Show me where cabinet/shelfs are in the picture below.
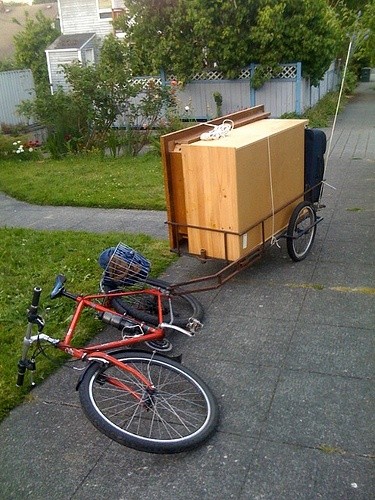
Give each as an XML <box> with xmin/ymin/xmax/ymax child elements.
<box><xmin>160</xmin><ymin>104</ymin><xmax>309</xmax><ymax>261</ymax></box>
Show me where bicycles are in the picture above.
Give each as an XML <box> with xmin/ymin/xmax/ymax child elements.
<box><xmin>16</xmin><ymin>241</ymin><xmax>223</xmax><ymax>457</ymax></box>
